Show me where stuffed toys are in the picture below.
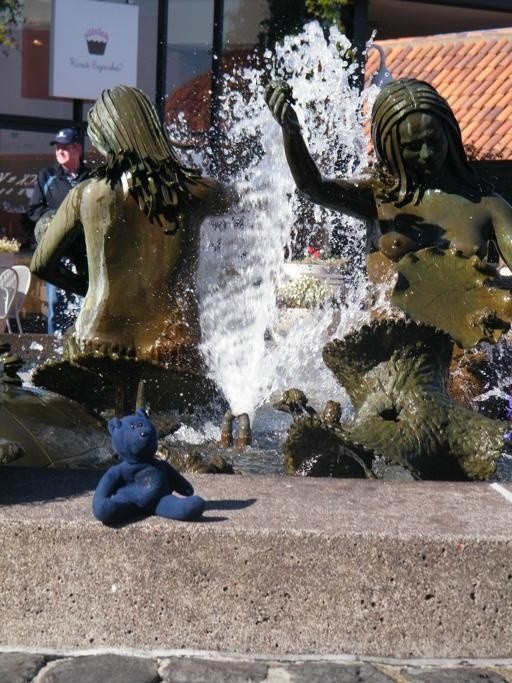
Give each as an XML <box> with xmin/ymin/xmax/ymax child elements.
<box><xmin>89</xmin><ymin>405</ymin><xmax>206</xmax><ymax>527</ymax></box>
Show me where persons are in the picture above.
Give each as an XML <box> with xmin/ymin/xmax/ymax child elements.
<box><xmin>24</xmin><ymin>126</ymin><xmax>100</xmax><ymax>335</ymax></box>
<box><xmin>28</xmin><ymin>82</ymin><xmax>235</xmax><ymax>373</ymax></box>
<box><xmin>261</xmin><ymin>66</ymin><xmax>511</xmax><ymax>320</ymax></box>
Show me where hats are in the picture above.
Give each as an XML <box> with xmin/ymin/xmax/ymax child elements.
<box><xmin>50</xmin><ymin>128</ymin><xmax>80</xmax><ymax>146</ymax></box>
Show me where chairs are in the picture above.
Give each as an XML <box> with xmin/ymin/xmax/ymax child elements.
<box><xmin>0</xmin><ymin>264</ymin><xmax>32</xmax><ymax>335</ymax></box>
